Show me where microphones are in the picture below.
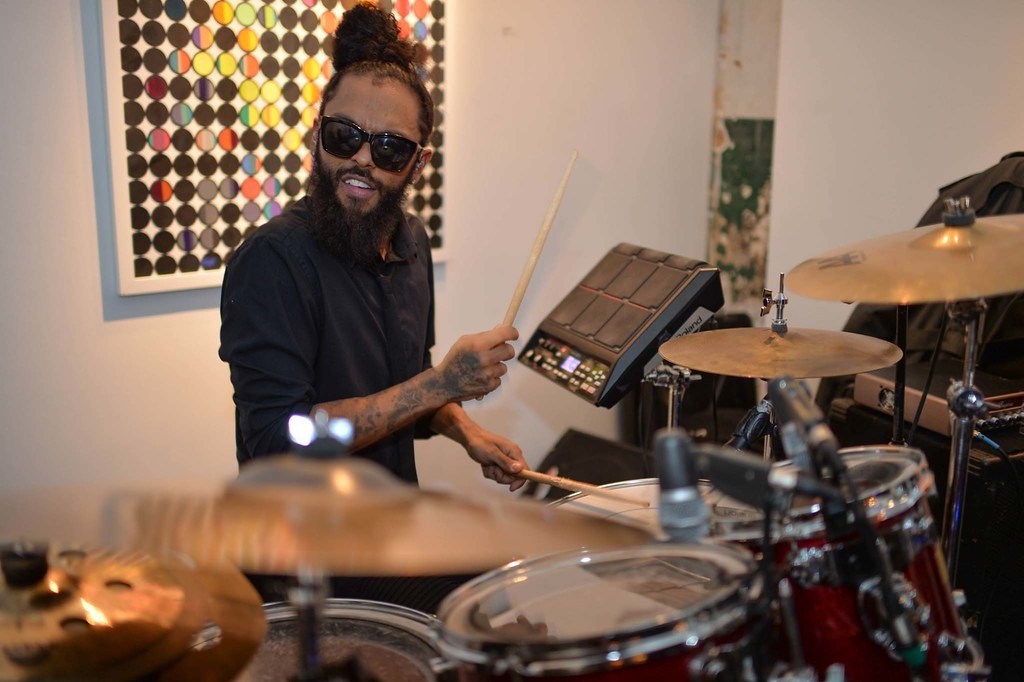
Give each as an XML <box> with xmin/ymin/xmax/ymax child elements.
<box><xmin>656</xmin><ymin>430</ymin><xmax>711</xmax><ymax>538</ymax></box>
<box><xmin>770</xmin><ymin>374</ymin><xmax>843</xmax><ymax>470</ymax></box>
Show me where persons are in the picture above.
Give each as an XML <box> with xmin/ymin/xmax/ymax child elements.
<box><xmin>217</xmin><ymin>0</ymin><xmax>533</xmax><ymax>499</ymax></box>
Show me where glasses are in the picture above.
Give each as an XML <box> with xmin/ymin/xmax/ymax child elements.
<box><xmin>320</xmin><ymin>116</ymin><xmax>420</xmax><ymax>173</ymax></box>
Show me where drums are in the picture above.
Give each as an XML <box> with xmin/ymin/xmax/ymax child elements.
<box><xmin>232</xmin><ymin>445</ymin><xmax>992</xmax><ymax>681</ymax></box>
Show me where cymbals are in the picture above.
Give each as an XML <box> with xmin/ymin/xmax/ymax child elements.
<box><xmin>0</xmin><ymin>440</ymin><xmax>653</xmax><ymax>576</ymax></box>
<box><xmin>658</xmin><ymin>327</ymin><xmax>902</xmax><ymax>377</ymax></box>
<box><xmin>785</xmin><ymin>213</ymin><xmax>1024</xmax><ymax>304</ymax></box>
<box><xmin>2</xmin><ymin>544</ymin><xmax>269</xmax><ymax>682</ymax></box>
